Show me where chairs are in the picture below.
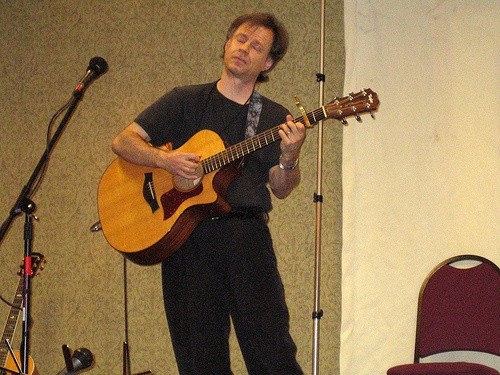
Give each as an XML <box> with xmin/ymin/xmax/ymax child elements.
<box><xmin>385</xmin><ymin>254</ymin><xmax>500</xmax><ymax>375</ymax></box>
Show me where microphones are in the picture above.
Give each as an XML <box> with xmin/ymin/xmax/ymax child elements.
<box><xmin>57</xmin><ymin>347</ymin><xmax>93</xmax><ymax>375</ymax></box>
<box><xmin>71</xmin><ymin>56</ymin><xmax>108</xmax><ymax>95</ymax></box>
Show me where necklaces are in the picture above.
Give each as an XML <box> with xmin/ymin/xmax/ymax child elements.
<box><xmin>192</xmin><ymin>75</ymin><xmax>255</xmax><ymax>135</ymax></box>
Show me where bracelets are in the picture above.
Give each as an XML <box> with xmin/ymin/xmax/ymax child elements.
<box><xmin>280</xmin><ymin>158</ymin><xmax>299</xmax><ymax>170</ymax></box>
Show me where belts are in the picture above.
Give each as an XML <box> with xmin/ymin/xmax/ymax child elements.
<box><xmin>233</xmin><ymin>210</ymin><xmax>260</xmax><ymax>219</ymax></box>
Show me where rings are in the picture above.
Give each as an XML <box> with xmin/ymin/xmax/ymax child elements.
<box><xmin>286</xmin><ymin>131</ymin><xmax>291</xmax><ymax>135</ymax></box>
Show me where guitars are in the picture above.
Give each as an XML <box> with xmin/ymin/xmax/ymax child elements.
<box><xmin>97</xmin><ymin>87</ymin><xmax>382</xmax><ymax>266</ymax></box>
<box><xmin>0</xmin><ymin>252</ymin><xmax>47</xmax><ymax>375</ymax></box>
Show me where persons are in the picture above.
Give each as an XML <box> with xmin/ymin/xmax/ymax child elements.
<box><xmin>111</xmin><ymin>13</ymin><xmax>304</xmax><ymax>375</ymax></box>
